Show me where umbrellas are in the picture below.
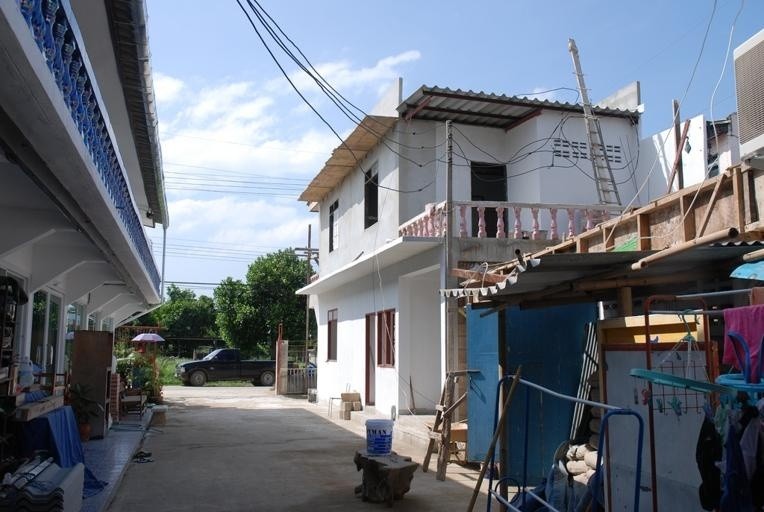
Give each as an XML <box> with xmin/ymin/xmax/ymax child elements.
<box><xmin>130</xmin><ymin>333</ymin><xmax>165</xmax><ymax>343</ymax></box>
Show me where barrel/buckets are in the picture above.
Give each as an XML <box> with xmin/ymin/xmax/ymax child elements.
<box><xmin>366</xmin><ymin>419</ymin><xmax>394</xmax><ymax>457</ymax></box>
<box><xmin>20</xmin><ymin>356</ymin><xmax>34</xmax><ymax>388</ymax></box>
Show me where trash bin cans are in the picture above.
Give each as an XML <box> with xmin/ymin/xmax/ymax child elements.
<box><xmin>290</xmin><ymin>364</ymin><xmax>301</xmax><ymax>395</ymax></box>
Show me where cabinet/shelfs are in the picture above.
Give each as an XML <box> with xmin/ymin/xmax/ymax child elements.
<box><xmin>69</xmin><ymin>329</ymin><xmax>115</xmax><ymax>441</ymax></box>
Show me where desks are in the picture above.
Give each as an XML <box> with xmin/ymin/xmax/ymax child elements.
<box><xmin>121</xmin><ymin>394</ymin><xmax>149</xmax><ymax>421</ymax></box>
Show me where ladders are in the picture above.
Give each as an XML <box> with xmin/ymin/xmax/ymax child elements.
<box><xmin>422</xmin><ymin>370</ymin><xmax>469</xmax><ymax>479</ymax></box>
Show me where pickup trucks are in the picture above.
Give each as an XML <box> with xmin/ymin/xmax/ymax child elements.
<box><xmin>174</xmin><ymin>348</ymin><xmax>300</xmax><ymax>387</ymax></box>
